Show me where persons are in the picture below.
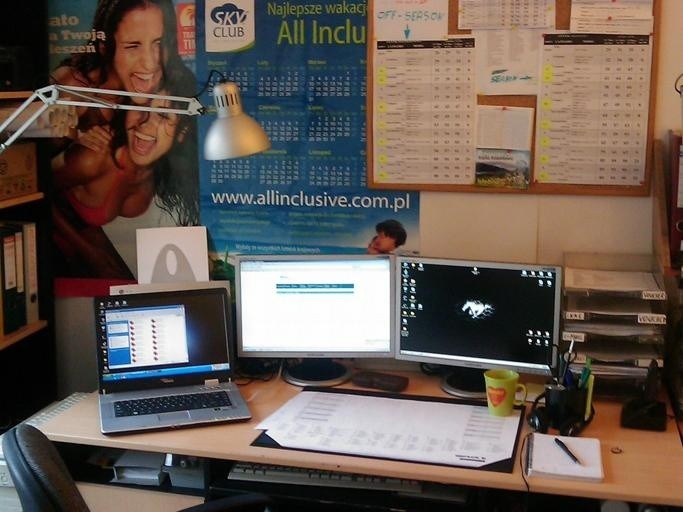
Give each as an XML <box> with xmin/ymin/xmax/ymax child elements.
<box><xmin>366</xmin><ymin>236</ymin><xmax>383</xmax><ymax>255</ymax></box>
<box><xmin>374</xmin><ymin>218</ymin><xmax>415</xmax><ymax>257</ymax></box>
<box><xmin>46</xmin><ymin>0</ymin><xmax>205</xmax><ymax>165</ymax></box>
<box><xmin>45</xmin><ymin>70</ymin><xmax>204</xmax><ymax>279</ymax></box>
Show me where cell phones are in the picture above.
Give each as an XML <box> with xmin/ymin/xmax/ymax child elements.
<box><xmin>351</xmin><ymin>370</ymin><xmax>409</xmax><ymax>392</ymax></box>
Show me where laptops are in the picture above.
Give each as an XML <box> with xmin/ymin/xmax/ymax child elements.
<box><xmin>94</xmin><ymin>286</ymin><xmax>253</xmax><ymax>436</ymax></box>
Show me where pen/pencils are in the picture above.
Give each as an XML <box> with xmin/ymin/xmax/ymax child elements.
<box><xmin>555</xmin><ymin>438</ymin><xmax>582</xmax><ymax>466</ymax></box>
<box><xmin>561</xmin><ymin>339</ymin><xmax>595</xmax><ymax>421</ymax></box>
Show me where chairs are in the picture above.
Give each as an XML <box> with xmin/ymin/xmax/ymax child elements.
<box><xmin>0</xmin><ymin>422</ymin><xmax>279</xmax><ymax>512</ymax></box>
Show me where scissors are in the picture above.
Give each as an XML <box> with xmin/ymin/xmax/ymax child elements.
<box><xmin>546</xmin><ymin>344</ymin><xmax>577</xmax><ymax>385</ymax></box>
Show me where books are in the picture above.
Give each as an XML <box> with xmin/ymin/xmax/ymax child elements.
<box><xmin>0</xmin><ymin>223</ymin><xmax>26</xmax><ymax>327</ymax></box>
<box><xmin>524</xmin><ymin>431</ymin><xmax>604</xmax><ymax>482</ymax></box>
<box><xmin>0</xmin><ymin>233</ymin><xmax>15</xmax><ymax>336</ymax></box>
<box><xmin>2</xmin><ymin>218</ymin><xmax>41</xmax><ymax>325</ymax></box>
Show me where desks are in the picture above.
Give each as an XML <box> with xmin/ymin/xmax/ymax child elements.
<box><xmin>34</xmin><ymin>367</ymin><xmax>683</xmax><ymax>512</ymax></box>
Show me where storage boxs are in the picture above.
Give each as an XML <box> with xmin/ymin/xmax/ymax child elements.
<box><xmin>0</xmin><ymin>141</ymin><xmax>40</xmax><ymax>202</ymax></box>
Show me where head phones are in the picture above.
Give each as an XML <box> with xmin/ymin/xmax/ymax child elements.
<box><xmin>527</xmin><ymin>390</ymin><xmax>596</xmax><ymax>437</ymax></box>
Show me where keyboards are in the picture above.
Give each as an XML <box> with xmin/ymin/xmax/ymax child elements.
<box><xmin>227</xmin><ymin>462</ymin><xmax>423</xmax><ymax>494</ymax></box>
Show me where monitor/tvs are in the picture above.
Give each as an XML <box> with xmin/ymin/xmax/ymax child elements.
<box><xmin>397</xmin><ymin>255</ymin><xmax>564</xmax><ymax>401</ymax></box>
<box><xmin>233</xmin><ymin>254</ymin><xmax>396</xmax><ymax>388</ymax></box>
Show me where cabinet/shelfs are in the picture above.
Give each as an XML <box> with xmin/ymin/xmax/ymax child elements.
<box><xmin>562</xmin><ymin>250</ymin><xmax>667</xmax><ymax>399</ymax></box>
<box><xmin>0</xmin><ymin>91</ymin><xmax>50</xmax><ymax>355</ymax></box>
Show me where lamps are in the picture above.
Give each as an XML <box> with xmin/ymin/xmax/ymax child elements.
<box><xmin>1</xmin><ymin>70</ymin><xmax>270</xmax><ymax>161</ymax></box>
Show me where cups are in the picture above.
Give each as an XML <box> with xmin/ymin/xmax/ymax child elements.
<box><xmin>481</xmin><ymin>368</ymin><xmax>526</xmax><ymax>416</ymax></box>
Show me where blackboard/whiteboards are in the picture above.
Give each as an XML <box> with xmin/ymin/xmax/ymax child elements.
<box><xmin>367</xmin><ymin>0</ymin><xmax>662</xmax><ymax>198</ymax></box>
